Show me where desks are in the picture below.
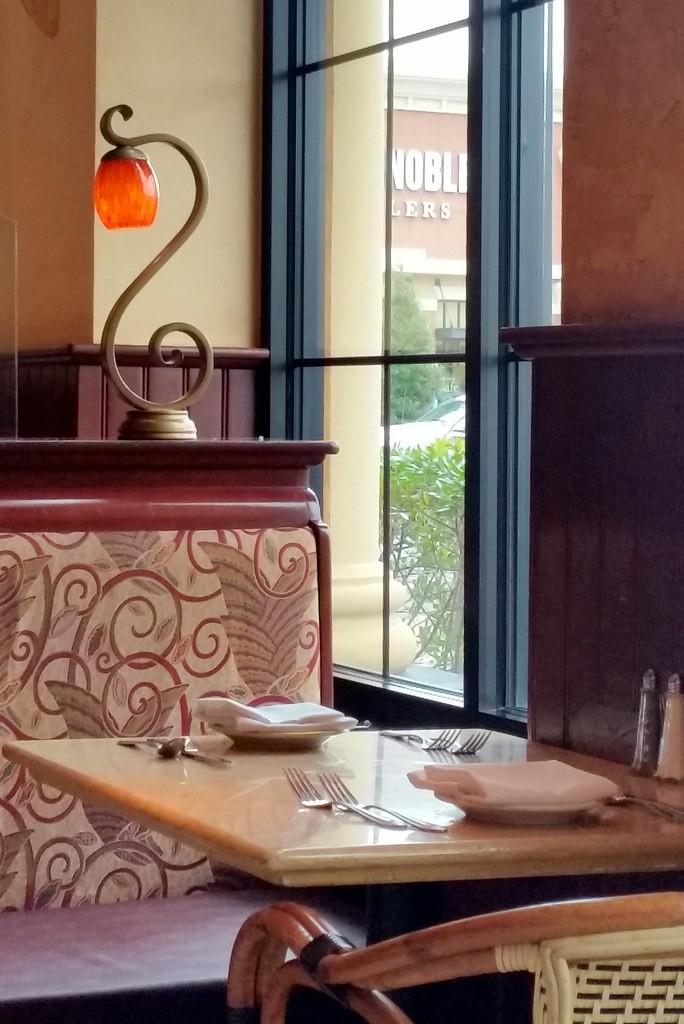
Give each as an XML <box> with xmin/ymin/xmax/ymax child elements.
<box><xmin>2</xmin><ymin>729</ymin><xmax>684</xmax><ymax>1024</ymax></box>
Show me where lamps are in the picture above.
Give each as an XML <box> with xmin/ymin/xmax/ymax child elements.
<box><xmin>92</xmin><ymin>105</ymin><xmax>213</xmax><ymax>439</ymax></box>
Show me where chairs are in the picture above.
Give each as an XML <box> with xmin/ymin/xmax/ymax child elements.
<box><xmin>226</xmin><ymin>890</ymin><xmax>684</xmax><ymax>1024</ymax></box>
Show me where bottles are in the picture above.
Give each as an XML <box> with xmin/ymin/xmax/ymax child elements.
<box><xmin>629</xmin><ymin>669</ymin><xmax>659</xmax><ymax>775</ymax></box>
<box><xmin>653</xmin><ymin>673</ymin><xmax>684</xmax><ymax>782</ymax></box>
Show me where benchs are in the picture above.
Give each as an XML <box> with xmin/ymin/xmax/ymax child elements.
<box><xmin>0</xmin><ymin>486</ymin><xmax>381</xmax><ymax>1024</ymax></box>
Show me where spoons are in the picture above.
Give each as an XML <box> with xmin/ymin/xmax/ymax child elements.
<box><xmin>117</xmin><ymin>737</ymin><xmax>191</xmax><ymax>757</ymax></box>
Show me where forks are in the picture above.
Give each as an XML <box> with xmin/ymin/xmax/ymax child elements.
<box><xmin>284</xmin><ymin>767</ymin><xmax>407</xmax><ymax>830</ymax></box>
<box><xmin>379</xmin><ymin>730</ymin><xmax>461</xmax><ymax>750</ymax></box>
<box><xmin>406</xmin><ymin>733</ymin><xmax>491</xmax><ymax>754</ymax></box>
<box><xmin>318</xmin><ymin>772</ymin><xmax>446</xmax><ymax>833</ymax></box>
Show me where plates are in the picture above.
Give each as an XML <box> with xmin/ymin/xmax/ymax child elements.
<box><xmin>207</xmin><ymin>722</ymin><xmax>350</xmax><ymax>748</ymax></box>
<box><xmin>433</xmin><ymin>790</ymin><xmax>602</xmax><ymax>825</ymax></box>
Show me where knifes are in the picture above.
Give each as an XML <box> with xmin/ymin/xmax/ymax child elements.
<box><xmin>148</xmin><ymin>738</ymin><xmax>231</xmax><ymax>764</ymax></box>
